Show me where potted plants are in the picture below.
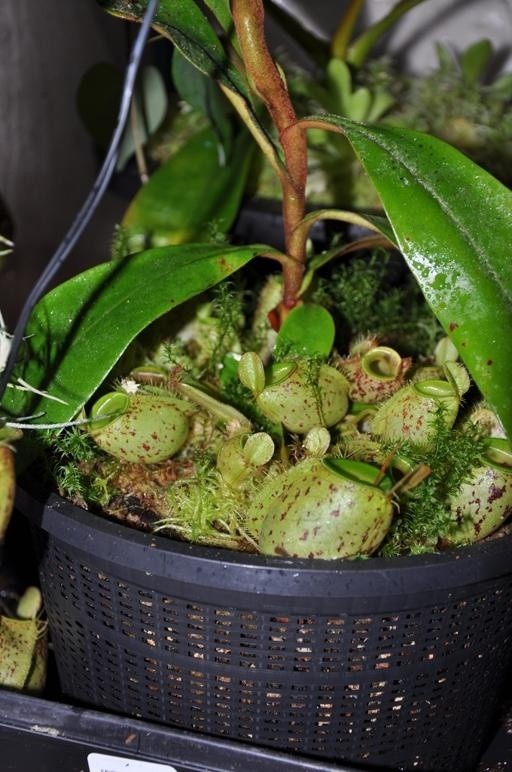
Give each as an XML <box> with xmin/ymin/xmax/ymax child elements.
<box><xmin>1</xmin><ymin>1</ymin><xmax>511</xmax><ymax>771</ymax></box>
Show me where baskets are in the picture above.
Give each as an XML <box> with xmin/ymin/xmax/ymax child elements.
<box><xmin>11</xmin><ymin>449</ymin><xmax>512</xmax><ymax>772</ymax></box>
